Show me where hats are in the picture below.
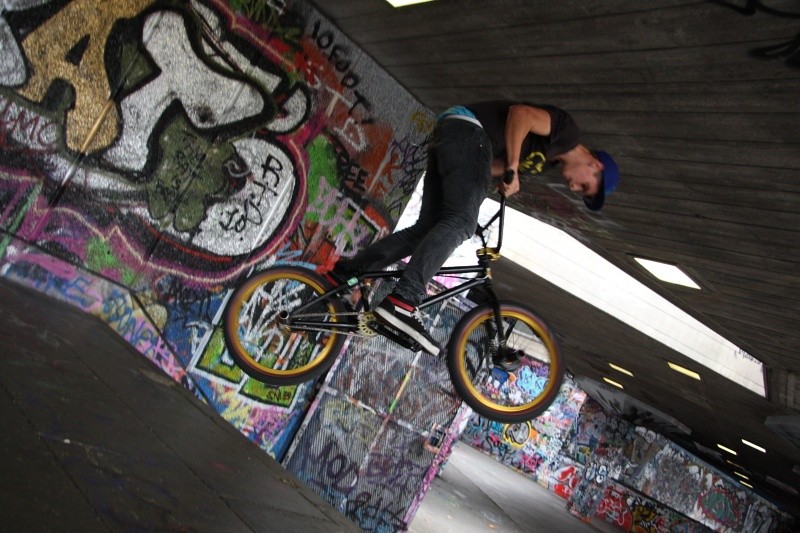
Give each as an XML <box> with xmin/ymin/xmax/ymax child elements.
<box><xmin>583</xmin><ymin>151</ymin><xmax>618</xmax><ymax>212</ymax></box>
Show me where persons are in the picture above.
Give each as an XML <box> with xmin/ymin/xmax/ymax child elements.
<box><xmin>325</xmin><ymin>99</ymin><xmax>619</xmax><ymax>355</ymax></box>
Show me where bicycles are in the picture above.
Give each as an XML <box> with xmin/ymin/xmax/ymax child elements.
<box><xmin>221</xmin><ymin>169</ymin><xmax>566</xmax><ymax>424</ymax></box>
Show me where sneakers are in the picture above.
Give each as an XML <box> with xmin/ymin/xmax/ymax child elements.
<box><xmin>314</xmin><ymin>272</ymin><xmax>354</xmax><ymax>306</ymax></box>
<box><xmin>373</xmin><ymin>293</ymin><xmax>441</xmax><ymax>357</ymax></box>
